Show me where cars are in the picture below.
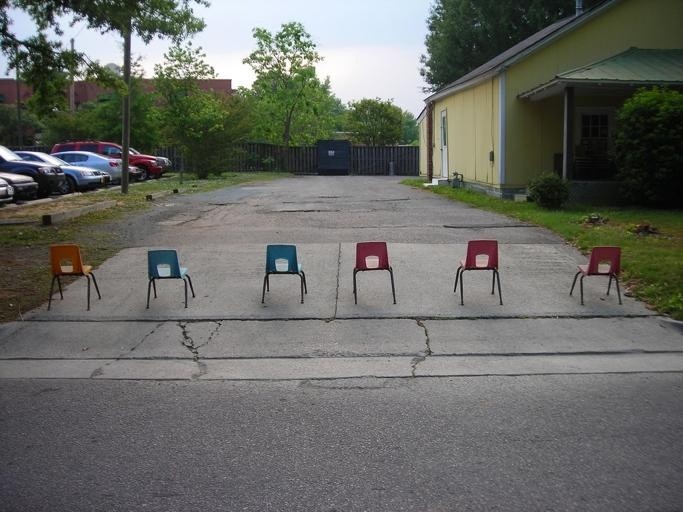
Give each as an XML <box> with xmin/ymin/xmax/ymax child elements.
<box><xmin>0</xmin><ymin>144</ymin><xmax>141</xmax><ymax>203</ymax></box>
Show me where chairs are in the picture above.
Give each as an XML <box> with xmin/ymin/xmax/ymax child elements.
<box><xmin>352</xmin><ymin>241</ymin><xmax>396</xmax><ymax>304</ymax></box>
<box><xmin>146</xmin><ymin>249</ymin><xmax>195</xmax><ymax>309</ymax></box>
<box><xmin>454</xmin><ymin>240</ymin><xmax>503</xmax><ymax>305</ymax></box>
<box><xmin>570</xmin><ymin>246</ymin><xmax>622</xmax><ymax>305</ymax></box>
<box><xmin>47</xmin><ymin>242</ymin><xmax>101</xmax><ymax>312</ymax></box>
<box><xmin>261</xmin><ymin>244</ymin><xmax>307</xmax><ymax>303</ymax></box>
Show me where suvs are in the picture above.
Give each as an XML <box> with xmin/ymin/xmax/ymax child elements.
<box><xmin>127</xmin><ymin>147</ymin><xmax>173</xmax><ymax>178</ymax></box>
<box><xmin>51</xmin><ymin>141</ymin><xmax>161</xmax><ymax>181</ymax></box>
